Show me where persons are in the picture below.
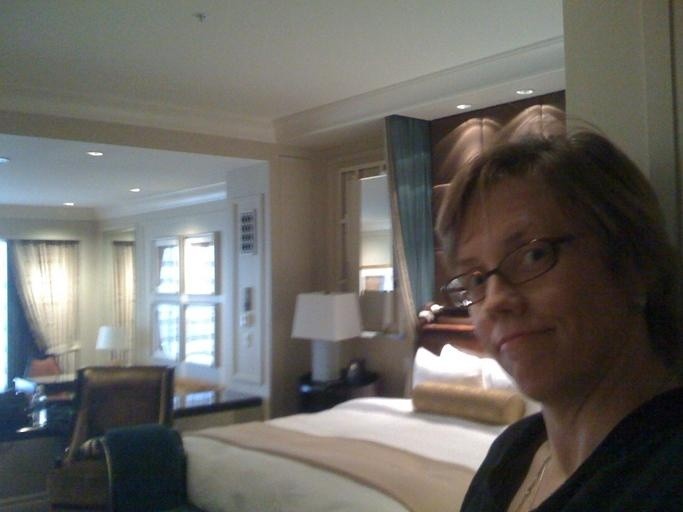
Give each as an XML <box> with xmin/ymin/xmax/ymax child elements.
<box><xmin>433</xmin><ymin>128</ymin><xmax>683</xmax><ymax>512</ymax></box>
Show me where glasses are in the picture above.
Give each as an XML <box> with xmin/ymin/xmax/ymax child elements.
<box><xmin>440</xmin><ymin>232</ymin><xmax>580</xmax><ymax>307</ymax></box>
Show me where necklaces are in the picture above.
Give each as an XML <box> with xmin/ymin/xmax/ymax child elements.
<box><xmin>515</xmin><ymin>456</ymin><xmax>554</xmax><ymax>512</ymax></box>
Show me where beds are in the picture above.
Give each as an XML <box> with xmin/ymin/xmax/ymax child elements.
<box><xmin>179</xmin><ymin>395</ymin><xmax>519</xmax><ymax>511</ymax></box>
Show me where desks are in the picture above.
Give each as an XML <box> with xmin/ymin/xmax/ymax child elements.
<box><xmin>10</xmin><ymin>374</ymin><xmax>76</xmax><ymax>427</ymax></box>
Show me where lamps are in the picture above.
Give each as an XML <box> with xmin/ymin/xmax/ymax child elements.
<box><xmin>94</xmin><ymin>323</ymin><xmax>131</xmax><ymax>362</ymax></box>
<box><xmin>289</xmin><ymin>291</ymin><xmax>363</xmax><ymax>384</ymax></box>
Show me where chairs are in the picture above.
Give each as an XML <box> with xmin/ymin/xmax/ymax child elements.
<box><xmin>99</xmin><ymin>421</ymin><xmax>186</xmax><ymax>512</ymax></box>
<box><xmin>43</xmin><ymin>363</ymin><xmax>187</xmax><ymax>512</ymax></box>
<box><xmin>21</xmin><ymin>354</ymin><xmax>76</xmax><ymax>422</ymax></box>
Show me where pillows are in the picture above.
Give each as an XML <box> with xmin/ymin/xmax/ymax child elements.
<box><xmin>412</xmin><ymin>342</ymin><xmax>541</xmax><ymax>419</ymax></box>
